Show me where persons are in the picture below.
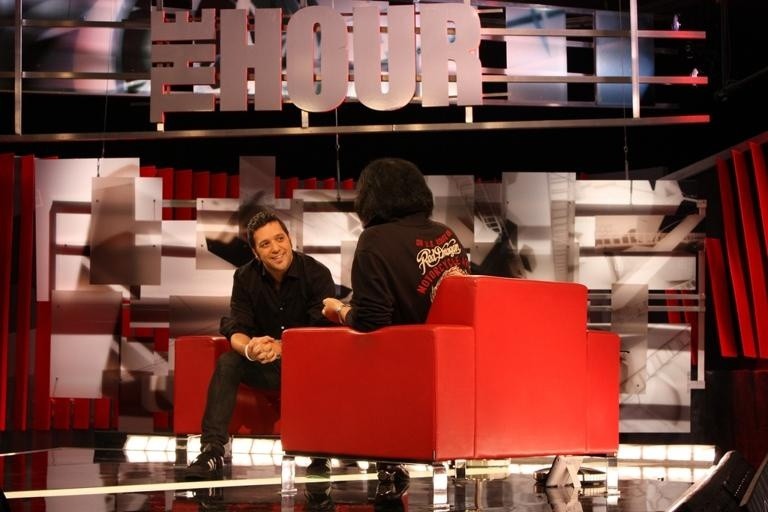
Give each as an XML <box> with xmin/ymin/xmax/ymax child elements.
<box><xmin>183</xmin><ymin>208</ymin><xmax>337</xmax><ymax>479</ymax></box>
<box><xmin>321</xmin><ymin>156</ymin><xmax>475</xmax><ymax>504</ymax></box>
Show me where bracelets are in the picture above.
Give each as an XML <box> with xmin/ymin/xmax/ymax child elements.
<box><xmin>337</xmin><ymin>304</ymin><xmax>352</xmax><ymax>328</ymax></box>
<box><xmin>244</xmin><ymin>344</ymin><xmax>255</xmax><ymax>362</ymax></box>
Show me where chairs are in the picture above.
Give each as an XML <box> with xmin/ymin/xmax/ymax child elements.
<box><xmin>174</xmin><ymin>334</ymin><xmax>280</xmax><ymax>467</ymax></box>
<box><xmin>279</xmin><ymin>274</ymin><xmax>620</xmax><ymax>512</ymax></box>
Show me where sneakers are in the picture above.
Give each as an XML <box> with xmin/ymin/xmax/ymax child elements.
<box><xmin>184</xmin><ymin>449</ymin><xmax>224</xmax><ymax>479</ymax></box>
<box><xmin>196</xmin><ymin>488</ymin><xmax>226</xmax><ymax>510</ymax></box>
<box><xmin>305</xmin><ymin>484</ymin><xmax>335</xmax><ymax>510</ymax></box>
<box><xmin>375</xmin><ymin>463</ymin><xmax>410</xmax><ymax>500</ymax></box>
<box><xmin>307</xmin><ymin>458</ymin><xmax>331</xmax><ymax>474</ymax></box>
